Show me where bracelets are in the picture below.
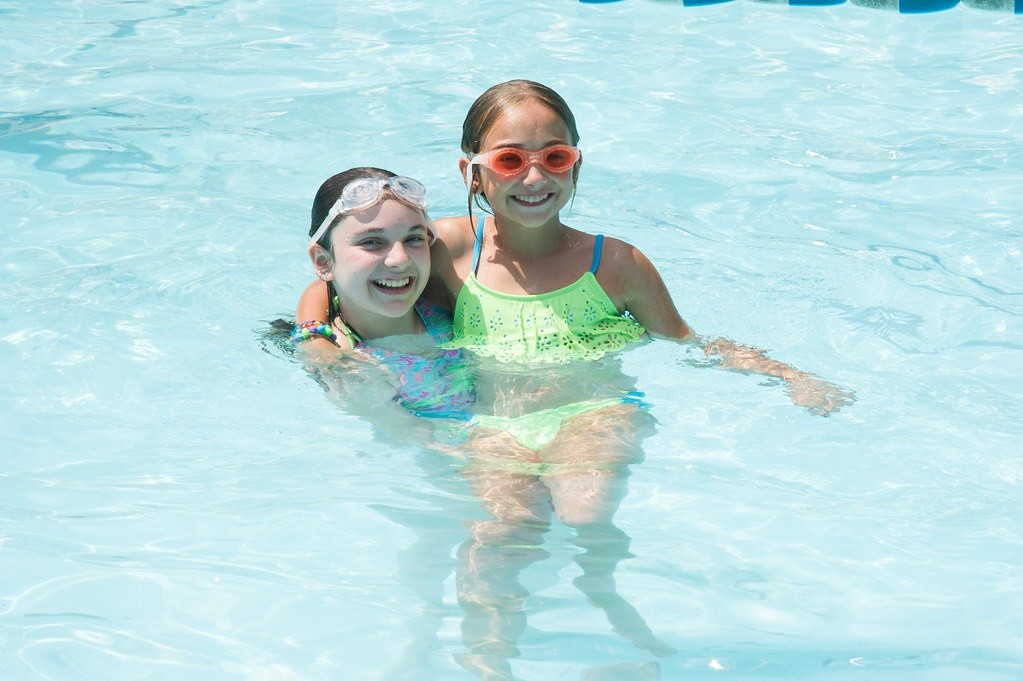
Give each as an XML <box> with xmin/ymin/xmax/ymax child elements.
<box><xmin>290</xmin><ymin>319</ymin><xmax>337</xmax><ymax>344</ymax></box>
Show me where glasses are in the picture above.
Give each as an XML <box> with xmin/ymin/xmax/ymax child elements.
<box><xmin>307</xmin><ymin>176</ymin><xmax>427</xmax><ymax>250</ymax></box>
<box><xmin>466</xmin><ymin>144</ymin><xmax>580</xmax><ymax>176</ymax></box>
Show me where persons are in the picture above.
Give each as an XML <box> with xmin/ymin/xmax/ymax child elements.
<box><xmin>296</xmin><ymin>78</ymin><xmax>687</xmax><ymax>357</ymax></box>
<box><xmin>307</xmin><ymin>169</ymin><xmax>454</xmax><ymax>349</ymax></box>
